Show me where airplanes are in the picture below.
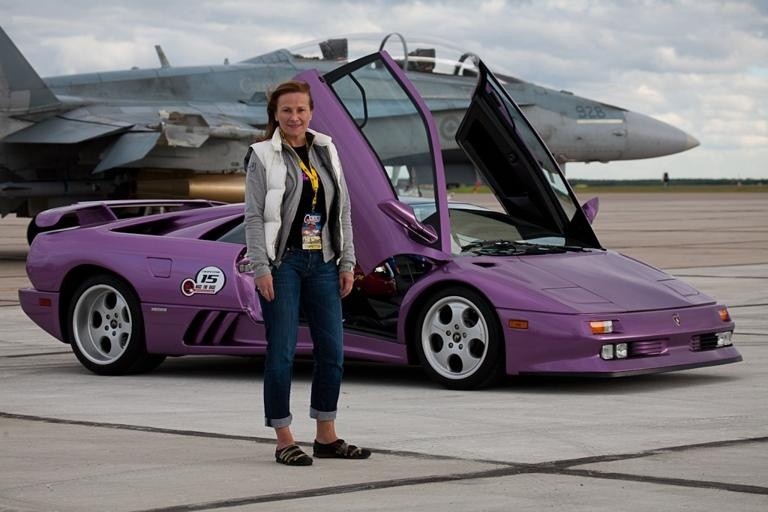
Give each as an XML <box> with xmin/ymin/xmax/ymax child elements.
<box><xmin>2</xmin><ymin>24</ymin><xmax>698</xmax><ymax>248</ymax></box>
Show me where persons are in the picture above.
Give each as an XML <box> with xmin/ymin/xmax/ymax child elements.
<box><xmin>663</xmin><ymin>172</ymin><xmax>669</xmax><ymax>188</ymax></box>
<box><xmin>242</xmin><ymin>81</ymin><xmax>371</xmax><ymax>466</ymax></box>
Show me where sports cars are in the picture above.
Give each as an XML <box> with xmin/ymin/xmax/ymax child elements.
<box><xmin>12</xmin><ymin>51</ymin><xmax>745</xmax><ymax>384</ymax></box>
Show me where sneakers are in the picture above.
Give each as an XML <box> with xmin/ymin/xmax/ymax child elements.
<box><xmin>276</xmin><ymin>445</ymin><xmax>312</xmax><ymax>466</ymax></box>
<box><xmin>314</xmin><ymin>438</ymin><xmax>370</xmax><ymax>460</ymax></box>
